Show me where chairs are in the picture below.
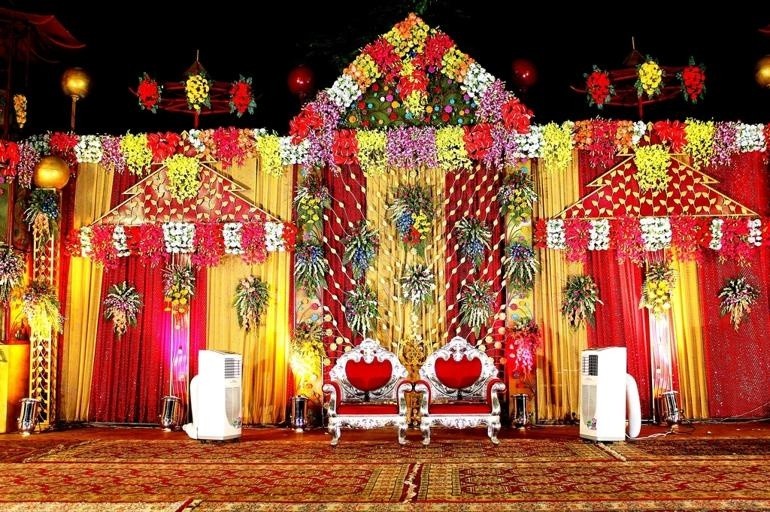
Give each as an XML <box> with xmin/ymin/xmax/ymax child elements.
<box><xmin>321</xmin><ymin>338</ymin><xmax>412</xmax><ymax>447</ymax></box>
<box><xmin>413</xmin><ymin>335</ymin><xmax>506</xmax><ymax>446</ymax></box>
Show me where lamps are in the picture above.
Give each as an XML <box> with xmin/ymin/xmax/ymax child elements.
<box><xmin>512</xmin><ymin>394</ymin><xmax>530</xmax><ymax>430</ymax></box>
<box><xmin>159</xmin><ymin>396</ymin><xmax>183</xmax><ymax>429</ymax></box>
<box><xmin>16</xmin><ymin>396</ymin><xmax>39</xmax><ymax>434</ymax></box>
<box><xmin>657</xmin><ymin>391</ymin><xmax>683</xmax><ymax>425</ymax></box>
<box><xmin>290</xmin><ymin>394</ymin><xmax>309</xmax><ymax>427</ymax></box>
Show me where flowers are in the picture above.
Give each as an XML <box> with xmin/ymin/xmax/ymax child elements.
<box><xmin>0</xmin><ymin>12</ymin><xmax>770</xmax><ymax>375</ymax></box>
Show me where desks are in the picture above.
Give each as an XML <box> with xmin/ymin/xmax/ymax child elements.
<box><xmin>1</xmin><ymin>343</ymin><xmax>30</xmax><ymax>434</ymax></box>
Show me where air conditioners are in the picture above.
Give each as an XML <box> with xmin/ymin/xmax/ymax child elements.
<box><xmin>579</xmin><ymin>344</ymin><xmax>643</xmax><ymax>442</ymax></box>
<box><xmin>188</xmin><ymin>349</ymin><xmax>244</xmax><ymax>440</ymax></box>
